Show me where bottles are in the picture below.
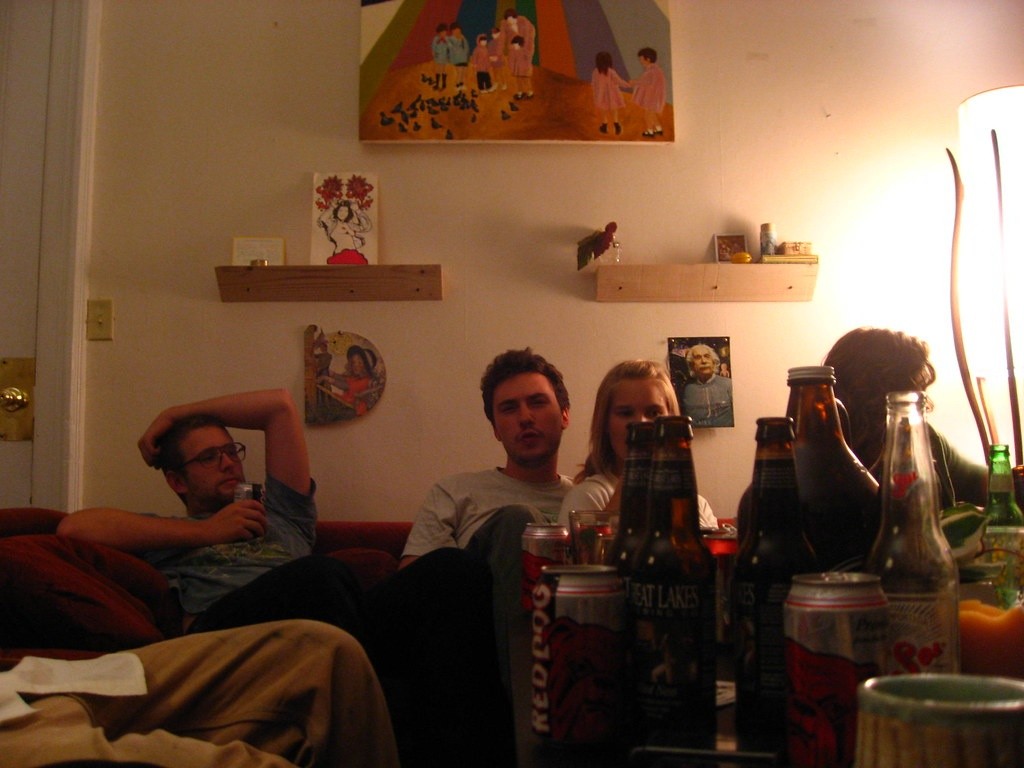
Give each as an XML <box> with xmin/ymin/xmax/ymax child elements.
<box><xmin>734</xmin><ymin>416</ymin><xmax>818</xmax><ymax>768</ymax></box>
<box><xmin>605</xmin><ymin>422</ymin><xmax>660</xmax><ymax>589</ymax></box>
<box><xmin>977</xmin><ymin>446</ymin><xmax>1024</xmax><ymax>610</ymax></box>
<box><xmin>620</xmin><ymin>414</ymin><xmax>718</xmax><ymax>768</ymax></box>
<box><xmin>857</xmin><ymin>390</ymin><xmax>961</xmax><ymax>676</ymax></box>
<box><xmin>784</xmin><ymin>366</ymin><xmax>883</xmax><ymax>574</ymax></box>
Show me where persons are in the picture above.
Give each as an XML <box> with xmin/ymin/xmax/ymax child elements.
<box><xmin>0</xmin><ymin>619</ymin><xmax>399</xmax><ymax>768</ymax></box>
<box><xmin>737</xmin><ymin>328</ymin><xmax>1024</xmax><ymax>543</ymax></box>
<box><xmin>683</xmin><ymin>345</ymin><xmax>733</xmax><ymax>426</ymax></box>
<box><xmin>56</xmin><ymin>388</ymin><xmax>518</xmax><ymax>768</ymax></box>
<box><xmin>560</xmin><ymin>359</ymin><xmax>719</xmax><ymax>529</ymax></box>
<box><xmin>401</xmin><ymin>347</ymin><xmax>573</xmax><ymax>690</ymax></box>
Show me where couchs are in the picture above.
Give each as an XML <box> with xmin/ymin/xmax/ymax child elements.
<box><xmin>0</xmin><ymin>507</ymin><xmax>737</xmax><ymax>707</ymax></box>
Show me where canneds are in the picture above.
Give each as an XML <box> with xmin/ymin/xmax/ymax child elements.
<box><xmin>520</xmin><ymin>521</ymin><xmax>572</xmax><ymax>630</ymax></box>
<box><xmin>567</xmin><ymin>521</ymin><xmax>612</xmax><ymax>566</ymax></box>
<box><xmin>234</xmin><ymin>482</ymin><xmax>265</xmax><ymax>505</ymax></box>
<box><xmin>781</xmin><ymin>570</ymin><xmax>890</xmax><ymax>768</ymax></box>
<box><xmin>530</xmin><ymin>564</ymin><xmax>627</xmax><ymax>752</ymax></box>
<box><xmin>701</xmin><ymin>528</ymin><xmax>745</xmax><ymax>649</ymax></box>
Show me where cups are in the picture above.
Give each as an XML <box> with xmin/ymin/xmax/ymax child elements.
<box><xmin>854</xmin><ymin>674</ymin><xmax>1024</xmax><ymax>768</ymax></box>
<box><xmin>567</xmin><ymin>509</ymin><xmax>620</xmax><ymax>565</ymax></box>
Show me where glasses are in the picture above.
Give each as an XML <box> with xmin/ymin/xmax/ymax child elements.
<box><xmin>166</xmin><ymin>442</ymin><xmax>246</xmax><ymax>471</ymax></box>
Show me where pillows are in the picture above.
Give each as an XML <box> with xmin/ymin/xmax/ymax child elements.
<box><xmin>0</xmin><ymin>536</ymin><xmax>165</xmax><ymax>649</ymax></box>
